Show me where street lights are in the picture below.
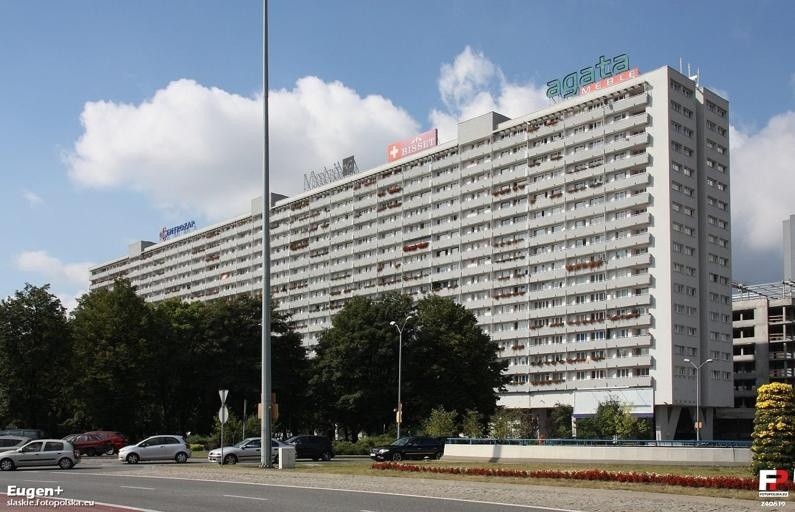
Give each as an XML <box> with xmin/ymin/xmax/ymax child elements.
<box><xmin>682</xmin><ymin>358</ymin><xmax>714</xmax><ymax>447</ymax></box>
<box><xmin>387</xmin><ymin>315</ymin><xmax>413</xmax><ymax>441</ymax></box>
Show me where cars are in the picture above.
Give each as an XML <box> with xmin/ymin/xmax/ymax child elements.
<box><xmin>205</xmin><ymin>434</ymin><xmax>299</xmax><ymax>467</ymax></box>
<box><xmin>116</xmin><ymin>432</ymin><xmax>191</xmax><ymax>465</ymax></box>
<box><xmin>0</xmin><ymin>426</ymin><xmax>131</xmax><ymax>474</ymax></box>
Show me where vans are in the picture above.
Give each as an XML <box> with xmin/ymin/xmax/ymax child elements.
<box><xmin>282</xmin><ymin>433</ymin><xmax>335</xmax><ymax>463</ymax></box>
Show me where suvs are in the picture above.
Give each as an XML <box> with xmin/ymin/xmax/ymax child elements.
<box><xmin>368</xmin><ymin>435</ymin><xmax>446</xmax><ymax>463</ymax></box>
